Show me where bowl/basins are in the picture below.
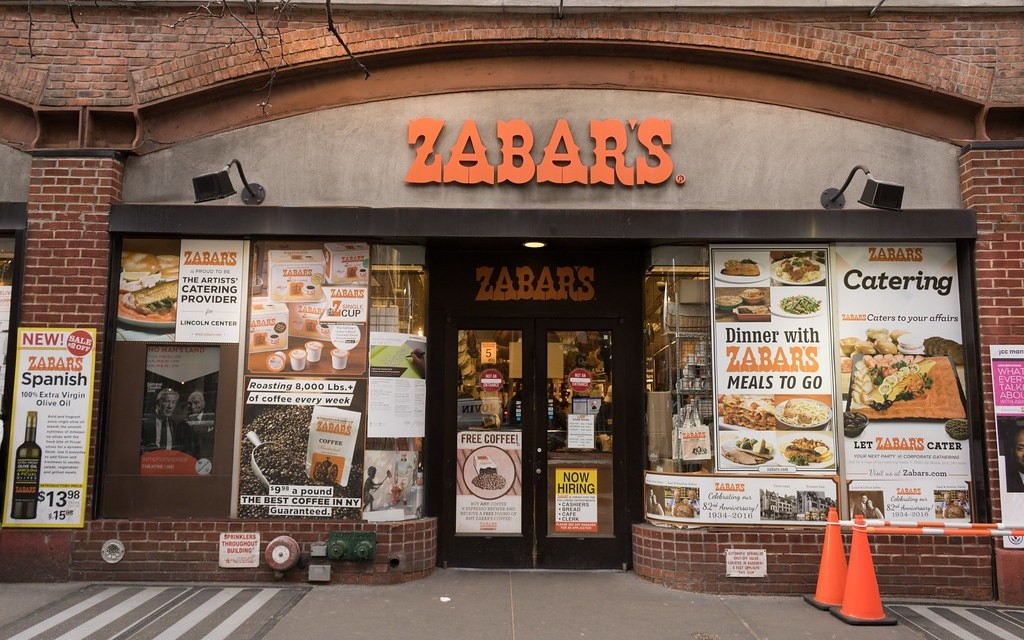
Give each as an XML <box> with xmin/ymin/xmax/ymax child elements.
<box><xmin>843</xmin><ymin>412</ymin><xmax>869</xmax><ymax>438</ymax></box>
<box><xmin>740</xmin><ymin>293</ymin><xmax>766</xmax><ymax>304</ymax></box>
<box><xmin>715</xmin><ymin>296</ymin><xmax>743</xmax><ymax>310</ymax></box>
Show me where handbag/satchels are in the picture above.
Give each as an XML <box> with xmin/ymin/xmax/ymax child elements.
<box><xmin>681</xmin><ymin>408</ymin><xmax>712</xmax><ymax>461</ymax></box>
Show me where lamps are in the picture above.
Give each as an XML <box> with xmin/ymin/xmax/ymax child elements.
<box><xmin>820</xmin><ymin>165</ymin><xmax>906</xmax><ymax>211</ymax></box>
<box><xmin>192</xmin><ymin>159</ymin><xmax>266</xmax><ymax>204</ymax></box>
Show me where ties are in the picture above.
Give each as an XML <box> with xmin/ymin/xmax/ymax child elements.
<box><xmin>159</xmin><ymin>418</ymin><xmax>167</xmax><ymax>450</ymax></box>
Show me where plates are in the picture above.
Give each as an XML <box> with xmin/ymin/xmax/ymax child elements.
<box><xmin>769</xmin><ymin>305</ymin><xmax>825</xmax><ymax>318</ymax></box>
<box><xmin>774</xmin><ymin>398</ymin><xmax>832</xmax><ymax>429</ymax></box>
<box><xmin>845</xmin><ymin>354</ymin><xmax>968</xmax><ymax>422</ymax></box>
<box><xmin>770</xmin><ymin>259</ymin><xmax>826</xmax><ymax>285</ymax></box>
<box><xmin>715</xmin><ymin>263</ymin><xmax>770</xmax><ymax>284</ymax></box>
<box><xmin>116</xmin><ymin>315</ymin><xmax>176</xmax><ymax>329</ymax></box>
<box><xmin>775</xmin><ymin>433</ymin><xmax>834</xmax><ymax>469</ymax></box>
<box><xmin>721</xmin><ymin>436</ymin><xmax>774</xmax><ymax>468</ymax></box>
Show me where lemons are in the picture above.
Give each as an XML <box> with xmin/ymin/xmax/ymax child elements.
<box><xmin>878</xmin><ymin>363</ymin><xmax>920</xmax><ymax>395</ymax></box>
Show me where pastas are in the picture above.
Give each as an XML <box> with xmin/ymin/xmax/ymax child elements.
<box><xmin>775</xmin><ymin>399</ymin><xmax>829</xmax><ymax>426</ymax></box>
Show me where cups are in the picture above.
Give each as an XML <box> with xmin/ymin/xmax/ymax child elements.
<box><xmin>304</xmin><ymin>341</ymin><xmax>324</xmax><ymax>363</ymax></box>
<box><xmin>355</xmin><ymin>268</ymin><xmax>367</xmax><ymax>280</ymax></box>
<box><xmin>316</xmin><ymin>323</ymin><xmax>331</xmax><ymax>335</ymax></box>
<box><xmin>268</xmin><ymin>335</ymin><xmax>280</xmax><ymax>346</ymax></box>
<box><xmin>289</xmin><ymin>349</ymin><xmax>308</xmax><ymax>371</ymax></box>
<box><xmin>897</xmin><ymin>334</ymin><xmax>926</xmax><ymax>354</ymax></box>
<box><xmin>302</xmin><ymin>284</ymin><xmax>316</xmax><ymax>297</ymax></box>
<box><xmin>265</xmin><ymin>351</ymin><xmax>287</xmax><ymax>372</ymax></box>
<box><xmin>329</xmin><ymin>348</ymin><xmax>350</xmax><ymax>370</ymax></box>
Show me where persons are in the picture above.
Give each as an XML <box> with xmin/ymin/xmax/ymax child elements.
<box><xmin>504</xmin><ymin>391</ymin><xmax>523</xmax><ymax>426</ymax></box>
<box><xmin>547</xmin><ymin>384</ymin><xmax>613</xmax><ymax>450</ymax></box>
<box><xmin>939</xmin><ymin>492</ymin><xmax>970</xmax><ymax>515</ymax></box>
<box><xmin>410</xmin><ymin>348</ymin><xmax>426</xmax><ymax>378</ymax></box>
<box><xmin>1004</xmin><ymin>425</ymin><xmax>1024</xmax><ymax>493</ymax></box>
<box><xmin>143</xmin><ymin>388</ymin><xmax>177</xmax><ymax>454</ymax></box>
<box><xmin>176</xmin><ymin>391</ymin><xmax>216</xmax><ymax>462</ymax></box>
<box><xmin>853</xmin><ymin>493</ymin><xmax>884</xmax><ymax>519</ymax></box>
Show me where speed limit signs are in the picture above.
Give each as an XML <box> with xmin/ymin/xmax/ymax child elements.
<box><xmin>480</xmin><ymin>342</ymin><xmax>497</xmax><ymax>364</ymax></box>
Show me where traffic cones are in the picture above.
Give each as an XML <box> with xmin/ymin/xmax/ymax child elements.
<box><xmin>802</xmin><ymin>506</ymin><xmax>849</xmax><ymax>610</ymax></box>
<box><xmin>828</xmin><ymin>515</ymin><xmax>899</xmax><ymax>626</ymax></box>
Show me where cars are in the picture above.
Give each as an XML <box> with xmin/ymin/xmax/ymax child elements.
<box><xmin>503</xmin><ymin>392</ymin><xmax>522</xmax><ymax>423</ymax></box>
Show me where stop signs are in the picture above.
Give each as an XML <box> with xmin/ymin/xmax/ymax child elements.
<box><xmin>568</xmin><ymin>369</ymin><xmax>592</xmax><ymax>392</ymax></box>
<box><xmin>479</xmin><ymin>370</ymin><xmax>504</xmax><ymax>393</ymax></box>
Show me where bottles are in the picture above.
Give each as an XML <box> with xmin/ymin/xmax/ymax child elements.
<box><xmin>10</xmin><ymin>410</ymin><xmax>42</xmax><ymax>520</ymax></box>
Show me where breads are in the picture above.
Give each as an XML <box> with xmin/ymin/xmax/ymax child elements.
<box><xmin>839</xmin><ymin>328</ymin><xmax>965</xmax><ymax>373</ymax></box>
<box><xmin>724</xmin><ymin>259</ymin><xmax>760</xmax><ymax>276</ymax></box>
<box><xmin>120</xmin><ymin>251</ymin><xmax>182</xmax><ymax>314</ymax></box>
